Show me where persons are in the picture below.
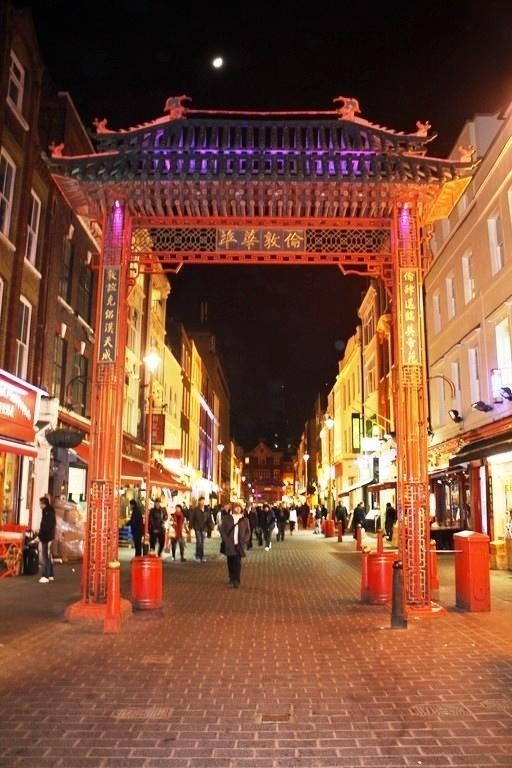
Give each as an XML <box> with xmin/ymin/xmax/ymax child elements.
<box><xmin>384</xmin><ymin>502</ymin><xmax>397</xmax><ymax>541</ymax></box>
<box><xmin>35</xmin><ymin>497</ymin><xmax>55</xmax><ymax>583</ymax></box>
<box><xmin>124</xmin><ymin>495</ymin><xmax>365</xmax><ymax>589</ymax></box>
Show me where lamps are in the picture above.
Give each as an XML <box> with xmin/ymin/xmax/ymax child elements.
<box><xmin>470</xmin><ymin>401</ymin><xmax>493</xmax><ymax>412</ymax></box>
<box><xmin>498</xmin><ymin>386</ymin><xmax>512</xmax><ymax>401</ymax></box>
<box><xmin>449</xmin><ymin>410</ymin><xmax>462</xmax><ymax>423</ymax></box>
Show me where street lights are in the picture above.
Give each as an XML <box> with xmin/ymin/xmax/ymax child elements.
<box><xmin>302</xmin><ymin>450</ymin><xmax>310</xmax><ymax>495</ymax></box>
<box><xmin>323</xmin><ymin>414</ymin><xmax>336</xmax><ymax>521</ymax></box>
<box><xmin>234</xmin><ymin>468</ymin><xmax>289</xmax><ymax>505</ymax></box>
<box><xmin>142</xmin><ymin>346</ymin><xmax>163</xmax><ymax>544</ymax></box>
<box><xmin>216</xmin><ymin>438</ymin><xmax>226</xmax><ymax>505</ymax></box>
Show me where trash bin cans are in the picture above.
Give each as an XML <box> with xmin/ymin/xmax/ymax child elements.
<box><xmin>325</xmin><ymin>520</ymin><xmax>335</xmax><ymax>537</ymax></box>
<box><xmin>361</xmin><ymin>550</ymin><xmax>399</xmax><ymax>605</ymax></box>
<box><xmin>130</xmin><ymin>557</ymin><xmax>165</xmax><ymax>609</ymax></box>
